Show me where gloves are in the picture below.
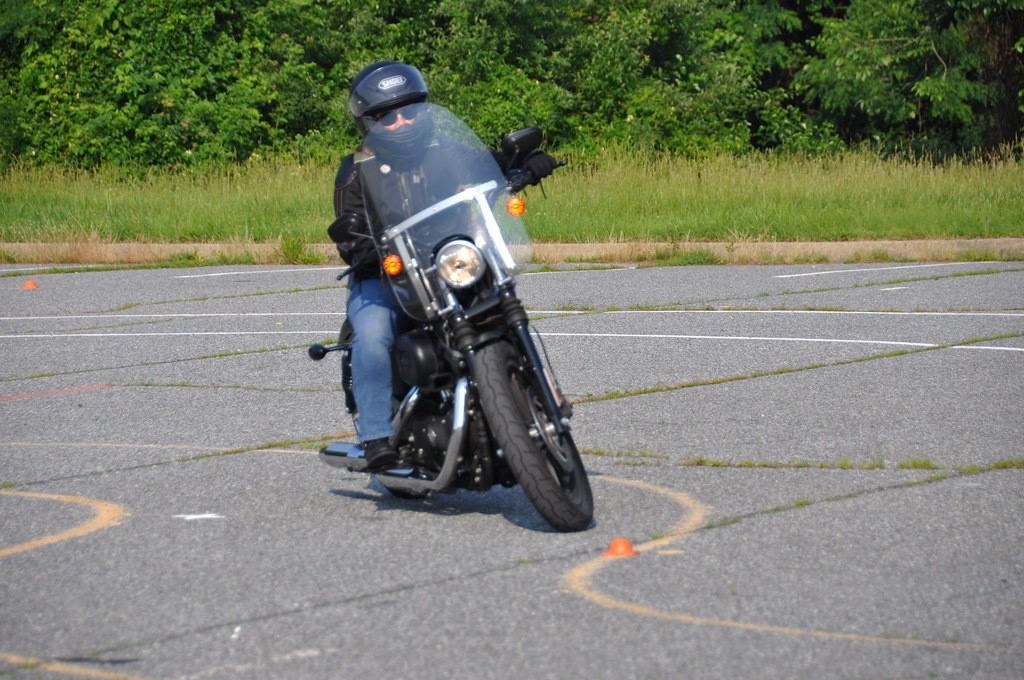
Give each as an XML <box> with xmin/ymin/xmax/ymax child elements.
<box><xmin>354</xmin><ymin>237</ymin><xmax>379</xmax><ymax>264</ymax></box>
<box><xmin>524</xmin><ymin>154</ymin><xmax>557</xmax><ymax>186</ymax></box>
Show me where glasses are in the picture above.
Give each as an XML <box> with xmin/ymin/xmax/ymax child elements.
<box><xmin>376</xmin><ymin>105</ymin><xmax>417</xmax><ymax>126</ymax></box>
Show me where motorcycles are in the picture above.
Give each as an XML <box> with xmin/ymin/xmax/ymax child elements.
<box><xmin>308</xmin><ymin>124</ymin><xmax>595</xmax><ymax>532</ymax></box>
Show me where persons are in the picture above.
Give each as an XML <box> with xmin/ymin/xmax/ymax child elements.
<box><xmin>333</xmin><ymin>61</ymin><xmax>559</xmax><ymax>472</ymax></box>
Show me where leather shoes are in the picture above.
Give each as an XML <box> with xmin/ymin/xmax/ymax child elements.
<box><xmin>364</xmin><ymin>438</ymin><xmax>398</xmax><ymax>470</ymax></box>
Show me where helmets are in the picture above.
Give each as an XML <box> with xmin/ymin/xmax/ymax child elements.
<box><xmin>348</xmin><ymin>60</ymin><xmax>434</xmax><ymax>170</ymax></box>
